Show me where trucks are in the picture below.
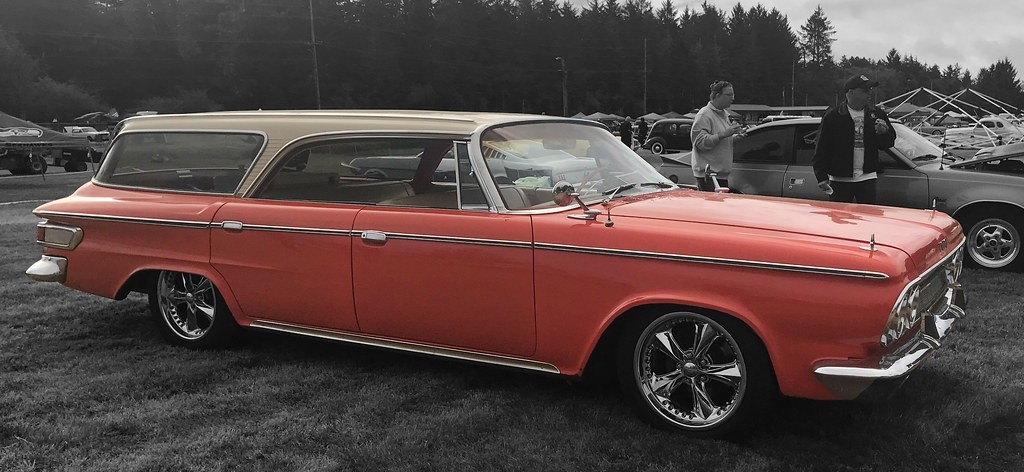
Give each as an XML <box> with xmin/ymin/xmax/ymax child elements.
<box><xmin>88</xmin><ymin>113</ymin><xmax>169</xmax><ymax>161</ymax></box>
<box><xmin>0</xmin><ymin>136</ymin><xmax>89</xmax><ymax>174</ymax></box>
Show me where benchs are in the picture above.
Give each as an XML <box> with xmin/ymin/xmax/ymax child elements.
<box><xmin>212</xmin><ymin>172</ymin><xmax>453</xmax><ymax>208</ymax></box>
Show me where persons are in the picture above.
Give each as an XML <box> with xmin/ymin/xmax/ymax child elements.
<box><xmin>690</xmin><ymin>80</ymin><xmax>748</xmax><ymax>193</ymax></box>
<box><xmin>637</xmin><ymin>118</ymin><xmax>648</xmax><ymax>147</ymax></box>
<box><xmin>620</xmin><ymin>116</ymin><xmax>632</xmax><ymax>148</ymax></box>
<box><xmin>813</xmin><ymin>74</ymin><xmax>896</xmax><ymax>204</ymax></box>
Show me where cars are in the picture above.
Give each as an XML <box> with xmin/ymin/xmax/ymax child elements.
<box><xmin>944</xmin><ymin>116</ymin><xmax>1024</xmax><ymax>137</ymax></box>
<box><xmin>642</xmin><ymin>118</ymin><xmax>695</xmax><ymax>155</ymax></box>
<box><xmin>24</xmin><ymin>110</ymin><xmax>969</xmax><ymax>435</ymax></box>
<box><xmin>63</xmin><ymin>126</ymin><xmax>111</xmax><ymax>142</ymax></box>
<box><xmin>903</xmin><ymin>111</ymin><xmax>961</xmax><ymax>135</ymax></box>
<box><xmin>658</xmin><ymin>117</ymin><xmax>1023</xmax><ymax>271</ymax></box>
<box><xmin>757</xmin><ymin>115</ymin><xmax>812</xmax><ymax>125</ymax></box>
<box><xmin>347</xmin><ymin>139</ymin><xmax>615</xmax><ymax>188</ymax></box>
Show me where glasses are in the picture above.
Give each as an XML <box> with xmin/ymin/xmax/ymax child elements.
<box><xmin>722</xmin><ymin>92</ymin><xmax>735</xmax><ymax>97</ymax></box>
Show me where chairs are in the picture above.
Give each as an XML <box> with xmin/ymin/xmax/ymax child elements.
<box><xmin>474</xmin><ymin>184</ymin><xmax>531</xmax><ymax>209</ymax></box>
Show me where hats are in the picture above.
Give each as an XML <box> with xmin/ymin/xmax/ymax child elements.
<box><xmin>844</xmin><ymin>73</ymin><xmax>880</xmax><ymax>93</ymax></box>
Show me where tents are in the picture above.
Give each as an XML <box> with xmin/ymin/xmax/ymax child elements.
<box><xmin>570</xmin><ymin>109</ymin><xmax>741</xmax><ymax>146</ymax></box>
<box><xmin>0</xmin><ymin>111</ymin><xmax>96</xmax><ymax>181</ymax></box>
<box><xmin>885</xmin><ymin>103</ymin><xmax>969</xmax><ymax>129</ymax></box>
